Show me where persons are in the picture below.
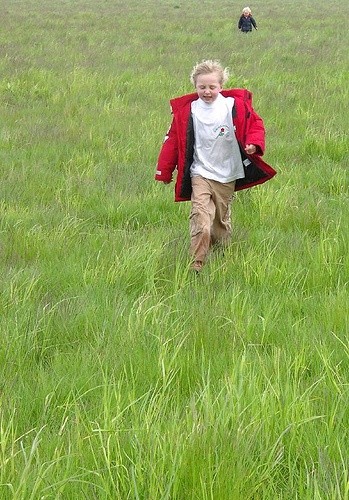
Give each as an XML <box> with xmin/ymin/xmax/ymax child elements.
<box><xmin>154</xmin><ymin>59</ymin><xmax>277</xmax><ymax>275</ymax></box>
<box><xmin>238</xmin><ymin>7</ymin><xmax>258</xmax><ymax>33</ymax></box>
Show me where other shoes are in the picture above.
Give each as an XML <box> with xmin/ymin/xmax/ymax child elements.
<box><xmin>189</xmin><ymin>261</ymin><xmax>204</xmax><ymax>275</ymax></box>
